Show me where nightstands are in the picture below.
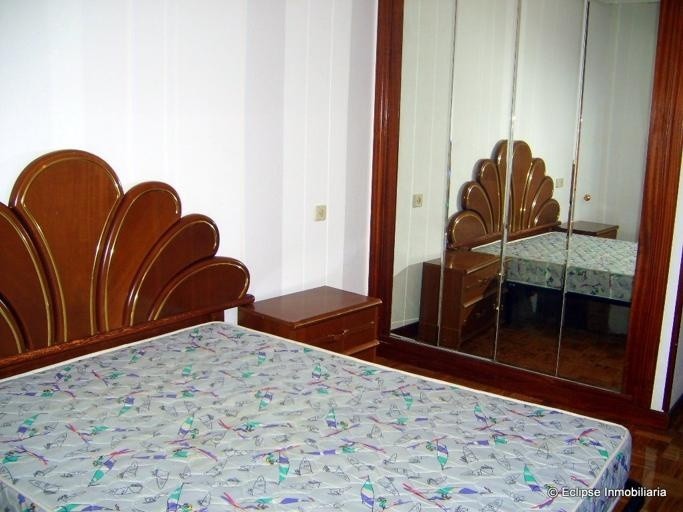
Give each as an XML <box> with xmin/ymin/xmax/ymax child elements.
<box><xmin>234</xmin><ymin>284</ymin><xmax>385</xmax><ymax>362</ymax></box>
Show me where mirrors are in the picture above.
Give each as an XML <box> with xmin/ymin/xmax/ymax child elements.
<box><xmin>390</xmin><ymin>0</ymin><xmax>661</xmax><ymax>395</ymax></box>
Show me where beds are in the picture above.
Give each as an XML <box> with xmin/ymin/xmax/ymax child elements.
<box><xmin>0</xmin><ymin>146</ymin><xmax>647</xmax><ymax>512</ymax></box>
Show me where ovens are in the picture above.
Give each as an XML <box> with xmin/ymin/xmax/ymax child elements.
<box><xmin>0</xmin><ymin>146</ymin><xmax>647</xmax><ymax>512</ymax></box>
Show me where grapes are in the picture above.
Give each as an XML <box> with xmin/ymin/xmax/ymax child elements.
<box><xmin>234</xmin><ymin>284</ymin><xmax>385</xmax><ymax>362</ymax></box>
